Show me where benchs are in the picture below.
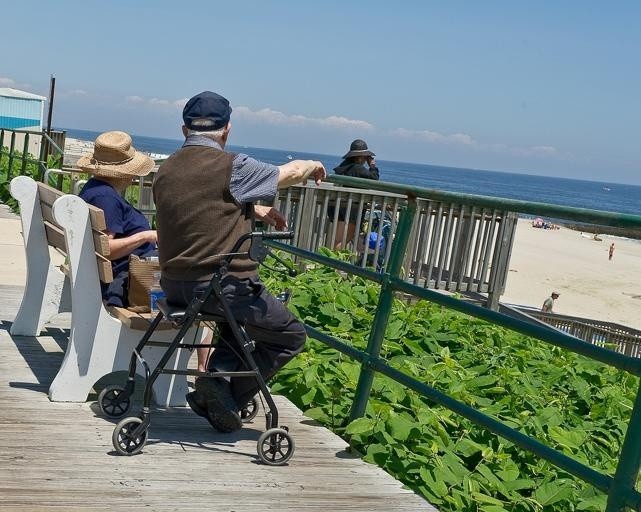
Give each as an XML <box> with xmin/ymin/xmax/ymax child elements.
<box><xmin>7</xmin><ymin>173</ymin><xmax>217</xmax><ymax>403</ymax></box>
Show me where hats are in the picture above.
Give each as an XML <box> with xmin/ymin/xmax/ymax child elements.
<box><xmin>342</xmin><ymin>139</ymin><xmax>376</xmax><ymax>159</ymax></box>
<box><xmin>183</xmin><ymin>91</ymin><xmax>232</xmax><ymax>131</ymax></box>
<box><xmin>77</xmin><ymin>130</ymin><xmax>155</xmax><ymax>177</ymax></box>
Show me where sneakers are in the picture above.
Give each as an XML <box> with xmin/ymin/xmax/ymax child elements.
<box><xmin>186</xmin><ymin>368</ymin><xmax>242</xmax><ymax>433</ymax></box>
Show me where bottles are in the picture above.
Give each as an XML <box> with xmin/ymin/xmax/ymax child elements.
<box><xmin>151</xmin><ymin>273</ymin><xmax>166</xmax><ymax>315</ymax></box>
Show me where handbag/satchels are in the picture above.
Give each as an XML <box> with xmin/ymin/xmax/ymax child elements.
<box><xmin>128</xmin><ymin>253</ymin><xmax>165</xmax><ymax>312</ymax></box>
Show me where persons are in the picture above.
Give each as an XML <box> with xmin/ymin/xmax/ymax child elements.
<box><xmin>609</xmin><ymin>243</ymin><xmax>614</xmax><ymax>259</ymax></box>
<box><xmin>153</xmin><ymin>92</ymin><xmax>327</xmax><ymax>432</ymax></box>
<box><xmin>77</xmin><ymin>130</ymin><xmax>215</xmax><ymax>373</ymax></box>
<box><xmin>328</xmin><ymin>139</ymin><xmax>379</xmax><ymax>253</ymax></box>
<box><xmin>543</xmin><ymin>291</ymin><xmax>560</xmax><ymax>313</ymax></box>
